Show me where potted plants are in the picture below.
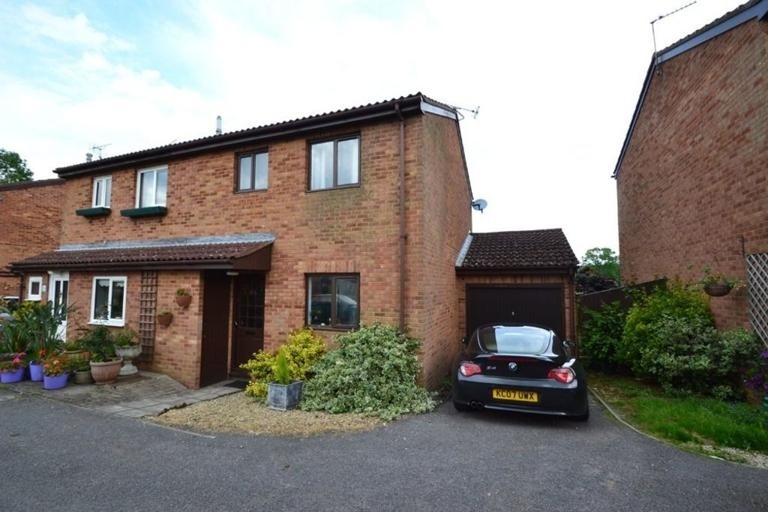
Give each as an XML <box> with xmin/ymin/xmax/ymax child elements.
<box><xmin>267</xmin><ymin>349</ymin><xmax>303</xmax><ymax>411</ymax></box>
<box><xmin>158</xmin><ymin>310</ymin><xmax>171</xmax><ymax>328</ymax></box>
<box><xmin>696</xmin><ymin>256</ymin><xmax>746</xmax><ymax>297</ymax></box>
<box><xmin>176</xmin><ymin>288</ymin><xmax>191</xmax><ymax>307</ymax></box>
<box><xmin>0</xmin><ymin>296</ymin><xmax>144</xmax><ymax>390</ymax></box>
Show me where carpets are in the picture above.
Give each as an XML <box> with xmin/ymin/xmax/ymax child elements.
<box><xmin>224</xmin><ymin>380</ymin><xmax>250</xmax><ymax>390</ymax></box>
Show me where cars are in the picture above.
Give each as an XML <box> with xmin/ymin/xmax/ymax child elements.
<box><xmin>451</xmin><ymin>322</ymin><xmax>592</xmax><ymax>423</ymax></box>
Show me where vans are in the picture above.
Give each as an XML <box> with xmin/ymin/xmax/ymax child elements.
<box><xmin>310</xmin><ymin>292</ymin><xmax>356</xmax><ymax>325</ymax></box>
